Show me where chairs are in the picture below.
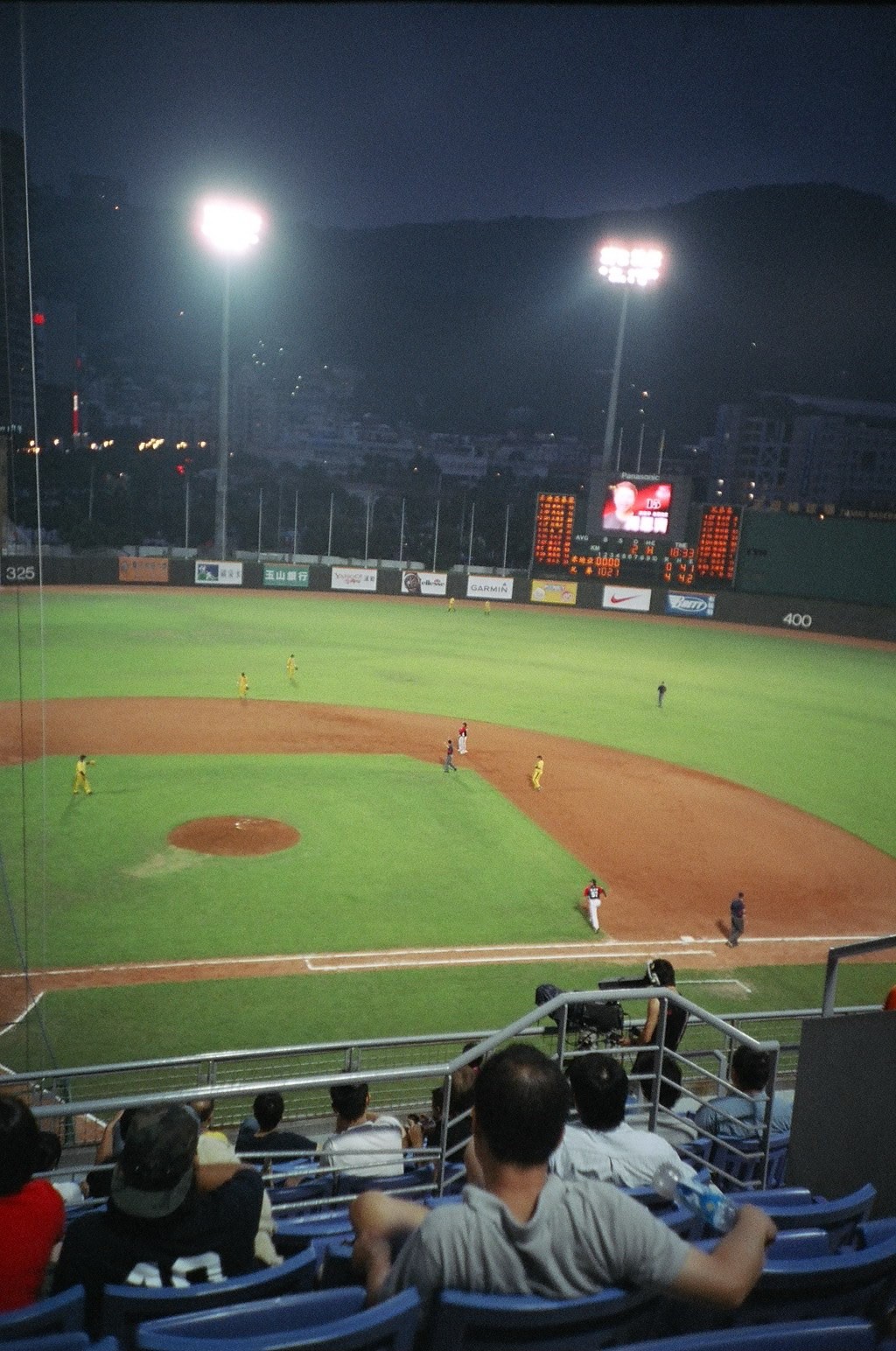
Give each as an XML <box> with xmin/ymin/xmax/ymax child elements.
<box><xmin>0</xmin><ymin>1094</ymin><xmax>896</xmax><ymax>1351</ymax></box>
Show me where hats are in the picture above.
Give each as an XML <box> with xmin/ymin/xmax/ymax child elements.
<box><xmin>111</xmin><ymin>1100</ymin><xmax>201</xmax><ymax>1218</ymax></box>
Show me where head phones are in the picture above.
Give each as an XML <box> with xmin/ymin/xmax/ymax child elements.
<box><xmin>648</xmin><ymin>960</ymin><xmax>660</xmax><ymax>987</ymax></box>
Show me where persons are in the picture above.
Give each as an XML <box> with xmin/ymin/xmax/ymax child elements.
<box><xmin>726</xmin><ymin>892</ymin><xmax>746</xmax><ymax>949</ymax></box>
<box><xmin>882</xmin><ymin>984</ymin><xmax>896</xmax><ymax>1011</ymax></box>
<box><xmin>445</xmin><ymin>739</ymin><xmax>457</xmax><ymax>773</ymax></box>
<box><xmin>72</xmin><ymin>754</ymin><xmax>96</xmax><ymax>797</ymax></box>
<box><xmin>630</xmin><ymin>958</ymin><xmax>691</xmax><ymax>1092</ymax></box>
<box><xmin>484</xmin><ymin>599</ymin><xmax>491</xmax><ymax>616</ymax></box>
<box><xmin>350</xmin><ymin>1044</ymin><xmax>777</xmax><ymax>1313</ymax></box>
<box><xmin>457</xmin><ymin>722</ymin><xmax>469</xmax><ymax>754</ymax></box>
<box><xmin>32</xmin><ymin>1043</ymin><xmax>793</xmax><ymax>1204</ymax></box>
<box><xmin>389</xmin><ymin>590</ymin><xmax>395</xmax><ymax>598</ymax></box>
<box><xmin>531</xmin><ymin>755</ymin><xmax>545</xmax><ymax>792</ymax></box>
<box><xmin>657</xmin><ymin>681</ymin><xmax>667</xmax><ymax>708</ymax></box>
<box><xmin>448</xmin><ymin>595</ymin><xmax>456</xmax><ymax>612</ymax></box>
<box><xmin>286</xmin><ymin>654</ymin><xmax>298</xmax><ymax>676</ymax></box>
<box><xmin>583</xmin><ymin>879</ymin><xmax>607</xmax><ymax>934</ymax></box>
<box><xmin>236</xmin><ymin>671</ymin><xmax>249</xmax><ymax>701</ymax></box>
<box><xmin>0</xmin><ymin>1090</ymin><xmax>285</xmax><ymax>1335</ymax></box>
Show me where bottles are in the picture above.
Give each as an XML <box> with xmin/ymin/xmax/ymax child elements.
<box><xmin>627</xmin><ymin>1092</ymin><xmax>640</xmax><ymax>1113</ymax></box>
<box><xmin>652</xmin><ymin>1163</ymin><xmax>738</xmax><ymax>1233</ymax></box>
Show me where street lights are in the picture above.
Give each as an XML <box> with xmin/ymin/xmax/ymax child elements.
<box><xmin>194</xmin><ymin>195</ymin><xmax>259</xmax><ymax>562</ymax></box>
<box><xmin>596</xmin><ymin>239</ymin><xmax>666</xmax><ymax>531</ymax></box>
